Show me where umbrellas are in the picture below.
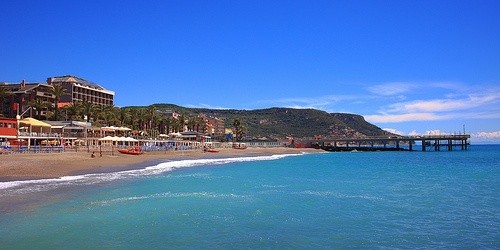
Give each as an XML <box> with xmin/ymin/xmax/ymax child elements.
<box><xmin>100</xmin><ymin>134</ymin><xmax>140</xmax><ymax>148</ymax></box>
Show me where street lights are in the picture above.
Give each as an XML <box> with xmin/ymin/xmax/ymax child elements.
<box><xmin>16</xmin><ymin>103</ymin><xmax>36</xmax><ymax>154</ymax></box>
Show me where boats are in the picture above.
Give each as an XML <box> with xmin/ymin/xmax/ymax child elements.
<box><xmin>232</xmin><ymin>143</ymin><xmax>245</xmax><ymax>149</ymax></box>
<box><xmin>203</xmin><ymin>146</ymin><xmax>219</xmax><ymax>153</ymax></box>
<box><xmin>118</xmin><ymin>147</ymin><xmax>143</xmax><ymax>156</ymax></box>
